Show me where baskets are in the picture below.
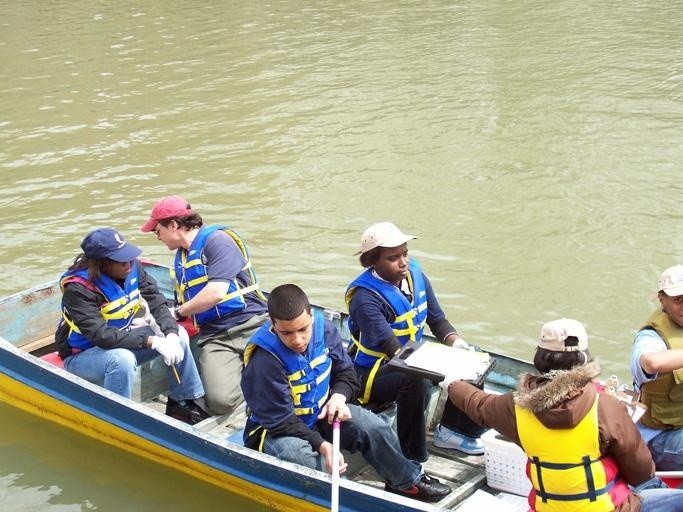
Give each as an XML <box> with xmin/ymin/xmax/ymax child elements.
<box><xmin>480</xmin><ymin>427</ymin><xmax>533</xmax><ymax>497</ymax></box>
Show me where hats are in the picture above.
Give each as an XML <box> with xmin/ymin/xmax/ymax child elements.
<box><xmin>140</xmin><ymin>195</ymin><xmax>192</xmax><ymax>233</ymax></box>
<box><xmin>352</xmin><ymin>222</ymin><xmax>417</xmax><ymax>257</ymax></box>
<box><xmin>535</xmin><ymin>317</ymin><xmax>588</xmax><ymax>365</ymax></box>
<box><xmin>80</xmin><ymin>228</ymin><xmax>141</xmax><ymax>262</ymax></box>
<box><xmin>657</xmin><ymin>264</ymin><xmax>683</xmax><ymax>297</ymax></box>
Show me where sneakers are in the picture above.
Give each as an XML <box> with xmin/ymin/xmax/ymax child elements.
<box><xmin>431</xmin><ymin>423</ymin><xmax>485</xmax><ymax>454</ymax></box>
<box><xmin>384</xmin><ymin>473</ymin><xmax>450</xmax><ymax>501</ymax></box>
<box><xmin>165</xmin><ymin>395</ymin><xmax>208</xmax><ymax>425</ymax></box>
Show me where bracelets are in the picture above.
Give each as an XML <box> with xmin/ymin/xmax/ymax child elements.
<box><xmin>174</xmin><ymin>308</ymin><xmax>187</xmax><ymax>322</ymax></box>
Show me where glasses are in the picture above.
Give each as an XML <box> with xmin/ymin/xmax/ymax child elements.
<box><xmin>153</xmin><ymin>229</ymin><xmax>160</xmax><ymax>235</ymax></box>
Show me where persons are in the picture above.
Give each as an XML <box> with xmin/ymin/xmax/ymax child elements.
<box><xmin>240</xmin><ymin>284</ymin><xmax>452</xmax><ymax>502</ymax></box>
<box><xmin>344</xmin><ymin>222</ymin><xmax>487</xmax><ymax>463</ymax></box>
<box><xmin>629</xmin><ymin>265</ymin><xmax>683</xmax><ymax>471</ymax></box>
<box><xmin>439</xmin><ymin>317</ymin><xmax>683</xmax><ymax>512</ymax></box>
<box><xmin>54</xmin><ymin>227</ymin><xmax>210</xmax><ymax>424</ymax></box>
<box><xmin>141</xmin><ymin>196</ymin><xmax>271</xmax><ymax>414</ymax></box>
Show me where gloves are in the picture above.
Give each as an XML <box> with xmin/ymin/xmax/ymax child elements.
<box><xmin>164</xmin><ymin>334</ymin><xmax>183</xmax><ymax>365</ymax></box>
<box><xmin>450</xmin><ymin>338</ymin><xmax>482</xmax><ymax>352</ymax></box>
<box><xmin>150</xmin><ymin>335</ymin><xmax>166</xmax><ymax>355</ymax></box>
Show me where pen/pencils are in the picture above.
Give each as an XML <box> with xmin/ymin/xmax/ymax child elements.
<box><xmin>171</xmin><ymin>365</ymin><xmax>180</xmax><ymax>385</ymax></box>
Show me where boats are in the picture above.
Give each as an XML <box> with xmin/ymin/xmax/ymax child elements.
<box><xmin>0</xmin><ymin>256</ymin><xmax>680</xmax><ymax>510</ymax></box>
